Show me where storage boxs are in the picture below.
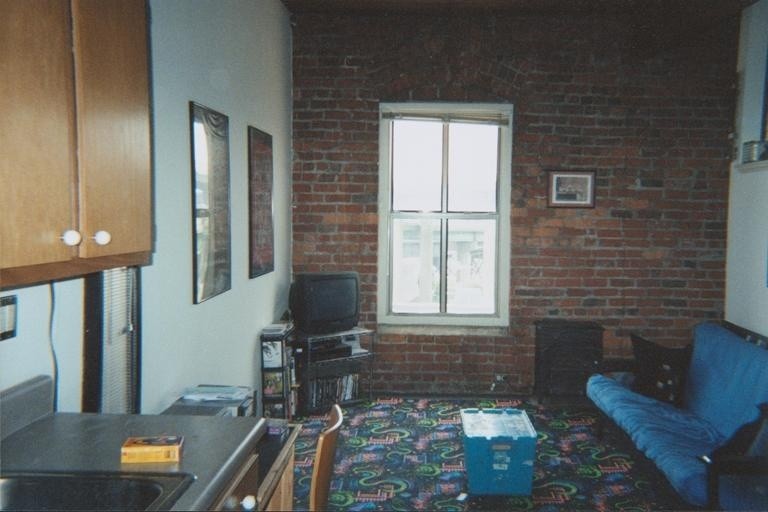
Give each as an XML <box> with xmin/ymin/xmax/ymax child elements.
<box><xmin>458</xmin><ymin>401</ymin><xmax>538</xmax><ymax>499</ymax></box>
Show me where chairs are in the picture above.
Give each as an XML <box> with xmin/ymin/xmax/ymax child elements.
<box><xmin>306</xmin><ymin>401</ymin><xmax>346</xmax><ymax>511</ymax></box>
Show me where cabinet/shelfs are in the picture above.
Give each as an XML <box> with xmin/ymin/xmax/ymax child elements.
<box><xmin>295</xmin><ymin>326</ymin><xmax>376</xmax><ymax>415</ymax></box>
<box><xmin>248</xmin><ymin>443</ymin><xmax>294</xmax><ymax>510</ymax></box>
<box><xmin>0</xmin><ymin>0</ymin><xmax>159</xmax><ymax>292</ymax></box>
<box><xmin>163</xmin><ymin>384</ymin><xmax>259</xmax><ymax>421</ymax></box>
<box><xmin>533</xmin><ymin>316</ymin><xmax>607</xmax><ymax>403</ymax></box>
<box><xmin>259</xmin><ymin>316</ymin><xmax>299</xmax><ymax>425</ymax></box>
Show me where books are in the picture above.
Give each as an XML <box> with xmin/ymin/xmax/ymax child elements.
<box><xmin>184</xmin><ymin>384</ymin><xmax>252</xmax><ymax>401</ymax></box>
<box><xmin>262</xmin><ymin>340</ymin><xmax>358</xmax><ymax>419</ymax></box>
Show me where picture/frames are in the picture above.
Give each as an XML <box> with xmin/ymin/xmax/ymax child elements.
<box><xmin>245</xmin><ymin>123</ymin><xmax>277</xmax><ymax>281</ymax></box>
<box><xmin>186</xmin><ymin>98</ymin><xmax>232</xmax><ymax>306</ymax></box>
<box><xmin>543</xmin><ymin>169</ymin><xmax>597</xmax><ymax>211</ymax></box>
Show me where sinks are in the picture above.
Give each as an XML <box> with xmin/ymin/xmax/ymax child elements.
<box><xmin>0</xmin><ymin>469</ymin><xmax>196</xmax><ymax>512</ymax></box>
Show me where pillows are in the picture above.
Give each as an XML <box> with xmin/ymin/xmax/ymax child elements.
<box><xmin>629</xmin><ymin>332</ymin><xmax>690</xmax><ymax>406</ymax></box>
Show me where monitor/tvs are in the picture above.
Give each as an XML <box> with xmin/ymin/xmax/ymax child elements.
<box><xmin>290</xmin><ymin>271</ymin><xmax>360</xmax><ymax>335</ymax></box>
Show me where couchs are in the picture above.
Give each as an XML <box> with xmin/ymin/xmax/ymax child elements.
<box><xmin>579</xmin><ymin>305</ymin><xmax>766</xmax><ymax>510</ymax></box>
